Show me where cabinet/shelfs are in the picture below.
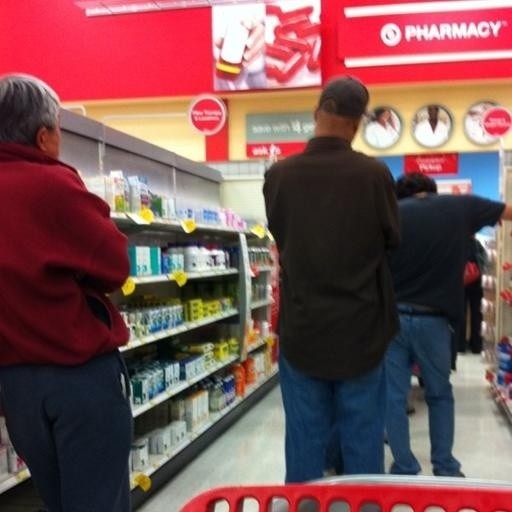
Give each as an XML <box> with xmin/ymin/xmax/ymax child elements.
<box><xmin>1</xmin><ymin>417</ymin><xmax>31</xmax><ymax>493</ymax></box>
<box><xmin>474</xmin><ymin>167</ymin><xmax>512</xmax><ymax>427</ymax></box>
<box><xmin>111</xmin><ymin>211</ymin><xmax>277</xmax><ymax>510</ymax></box>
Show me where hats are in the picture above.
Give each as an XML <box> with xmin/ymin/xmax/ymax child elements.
<box><xmin>318</xmin><ymin>78</ymin><xmax>369</xmax><ymax>118</ymax></box>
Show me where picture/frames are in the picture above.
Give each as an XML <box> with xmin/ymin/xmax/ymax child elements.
<box><xmin>462</xmin><ymin>98</ymin><xmax>506</xmax><ymax>146</ymax></box>
<box><xmin>410</xmin><ymin>103</ymin><xmax>455</xmax><ymax>149</ymax></box>
<box><xmin>362</xmin><ymin>105</ymin><xmax>402</xmax><ymax>150</ymax></box>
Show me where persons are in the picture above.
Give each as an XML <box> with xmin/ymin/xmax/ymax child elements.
<box><xmin>457</xmin><ymin>240</ymin><xmax>487</xmax><ymax>353</ymax></box>
<box><xmin>0</xmin><ymin>71</ymin><xmax>134</xmax><ymax>510</ymax></box>
<box><xmin>414</xmin><ymin>106</ymin><xmax>447</xmax><ymax>145</ymax></box>
<box><xmin>258</xmin><ymin>73</ymin><xmax>402</xmax><ymax>488</ymax></box>
<box><xmin>384</xmin><ymin>172</ymin><xmax>512</xmax><ymax>478</ymax></box>
<box><xmin>364</xmin><ymin>107</ymin><xmax>399</xmax><ymax>148</ymax></box>
